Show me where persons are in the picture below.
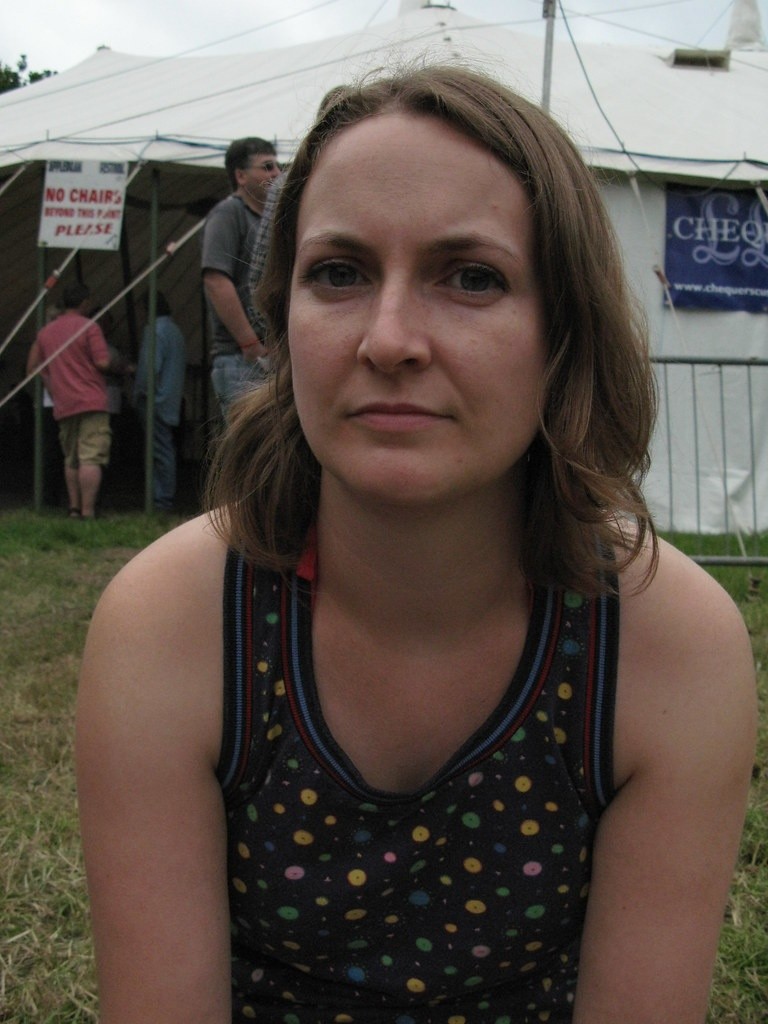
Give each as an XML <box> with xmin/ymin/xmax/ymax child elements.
<box><xmin>74</xmin><ymin>61</ymin><xmax>757</xmax><ymax>1024</ymax></box>
<box><xmin>26</xmin><ymin>88</ymin><xmax>358</xmax><ymax>514</ymax></box>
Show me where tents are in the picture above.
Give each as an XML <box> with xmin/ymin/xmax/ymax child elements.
<box><xmin>2</xmin><ymin>0</ymin><xmax>764</xmax><ymax>536</ymax></box>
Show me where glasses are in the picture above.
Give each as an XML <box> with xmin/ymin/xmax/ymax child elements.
<box><xmin>237</xmin><ymin>161</ymin><xmax>280</xmax><ymax>171</ymax></box>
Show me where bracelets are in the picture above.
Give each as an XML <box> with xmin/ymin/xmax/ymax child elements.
<box><xmin>240</xmin><ymin>340</ymin><xmax>261</xmax><ymax>349</ymax></box>
